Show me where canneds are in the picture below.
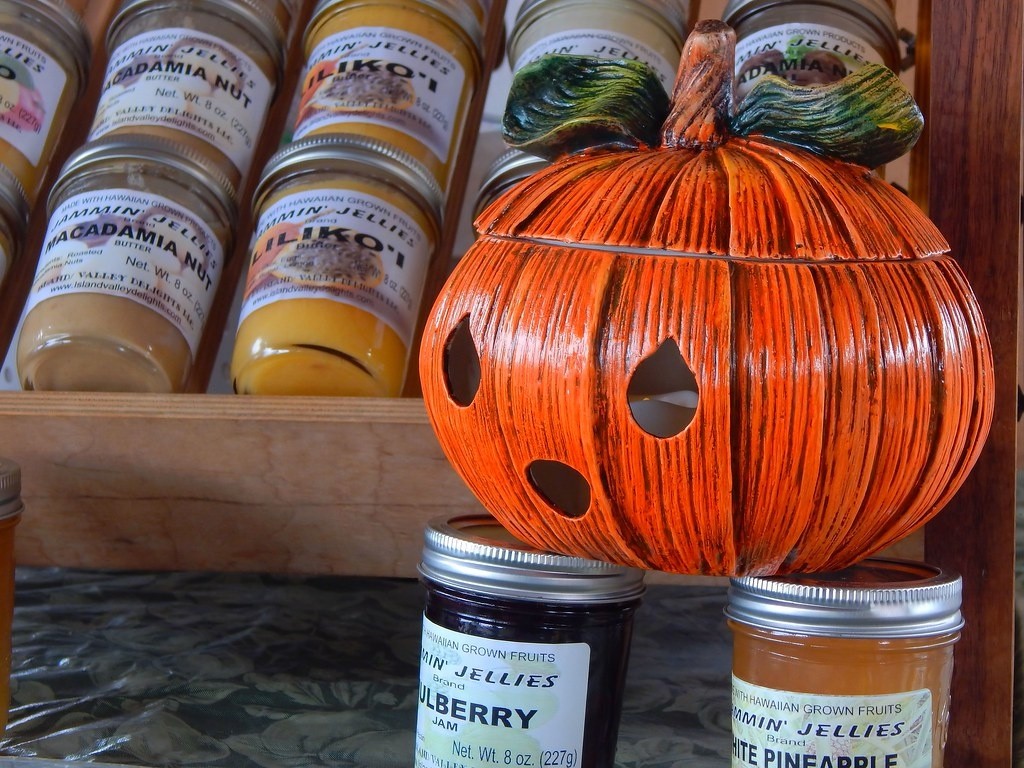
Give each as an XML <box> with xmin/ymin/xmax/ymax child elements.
<box><xmin>0</xmin><ymin>0</ymin><xmax>484</xmax><ymax>396</ymax></box>
<box><xmin>0</xmin><ymin>454</ymin><xmax>25</xmax><ymax>741</ymax></box>
<box><xmin>412</xmin><ymin>512</ymin><xmax>967</xmax><ymax>768</ymax></box>
<box><xmin>471</xmin><ymin>0</ymin><xmax>899</xmax><ymax>239</ymax></box>
